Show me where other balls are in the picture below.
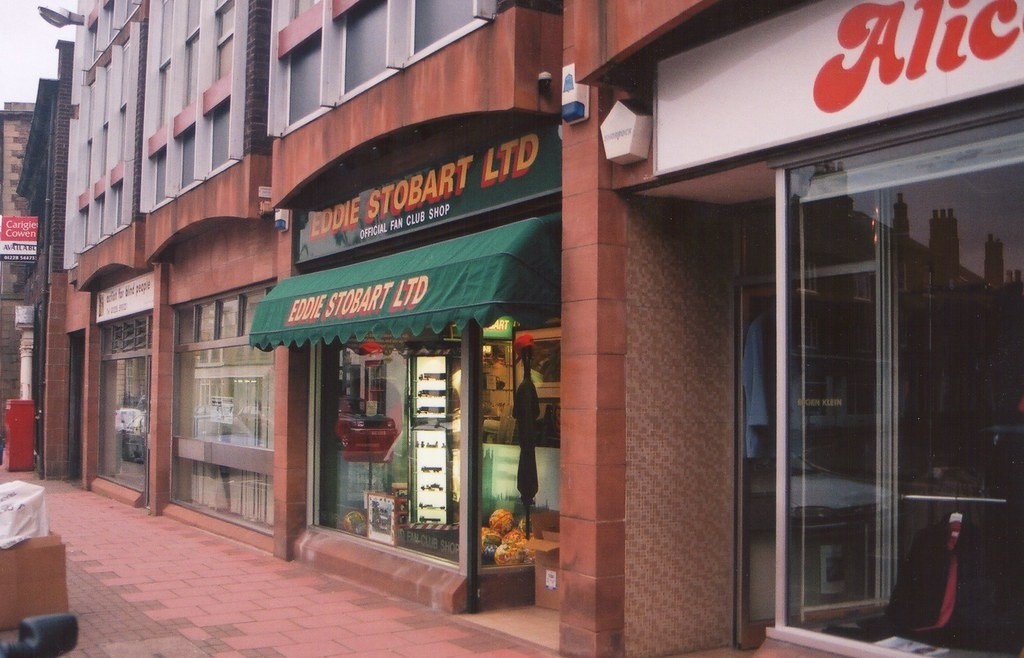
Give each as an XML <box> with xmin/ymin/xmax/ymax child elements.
<box><xmin>343</xmin><ymin>510</ymin><xmax>366</xmax><ymax>534</ymax></box>
<box><xmin>482</xmin><ymin>507</ymin><xmax>535</xmax><ymax>567</ymax></box>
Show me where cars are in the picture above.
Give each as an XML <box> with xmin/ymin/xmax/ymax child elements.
<box><xmin>117</xmin><ymin>415</ymin><xmax>147</xmax><ymax>463</ymax></box>
<box><xmin>195</xmin><ymin>404</ymin><xmax>238</xmax><ymax>436</ymax></box>
<box><xmin>235</xmin><ymin>404</ymin><xmax>273</xmax><ymax>436</ymax></box>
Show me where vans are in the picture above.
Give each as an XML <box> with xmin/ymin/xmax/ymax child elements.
<box><xmin>380</xmin><ymin>368</ymin><xmax>461</xmax><ymax>500</ymax></box>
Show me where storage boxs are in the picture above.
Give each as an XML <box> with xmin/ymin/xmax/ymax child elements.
<box><xmin>0</xmin><ymin>531</ymin><xmax>68</xmax><ymax>632</ymax></box>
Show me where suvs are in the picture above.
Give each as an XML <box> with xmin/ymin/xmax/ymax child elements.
<box><xmin>335</xmin><ymin>396</ymin><xmax>398</xmax><ymax>450</ymax></box>
